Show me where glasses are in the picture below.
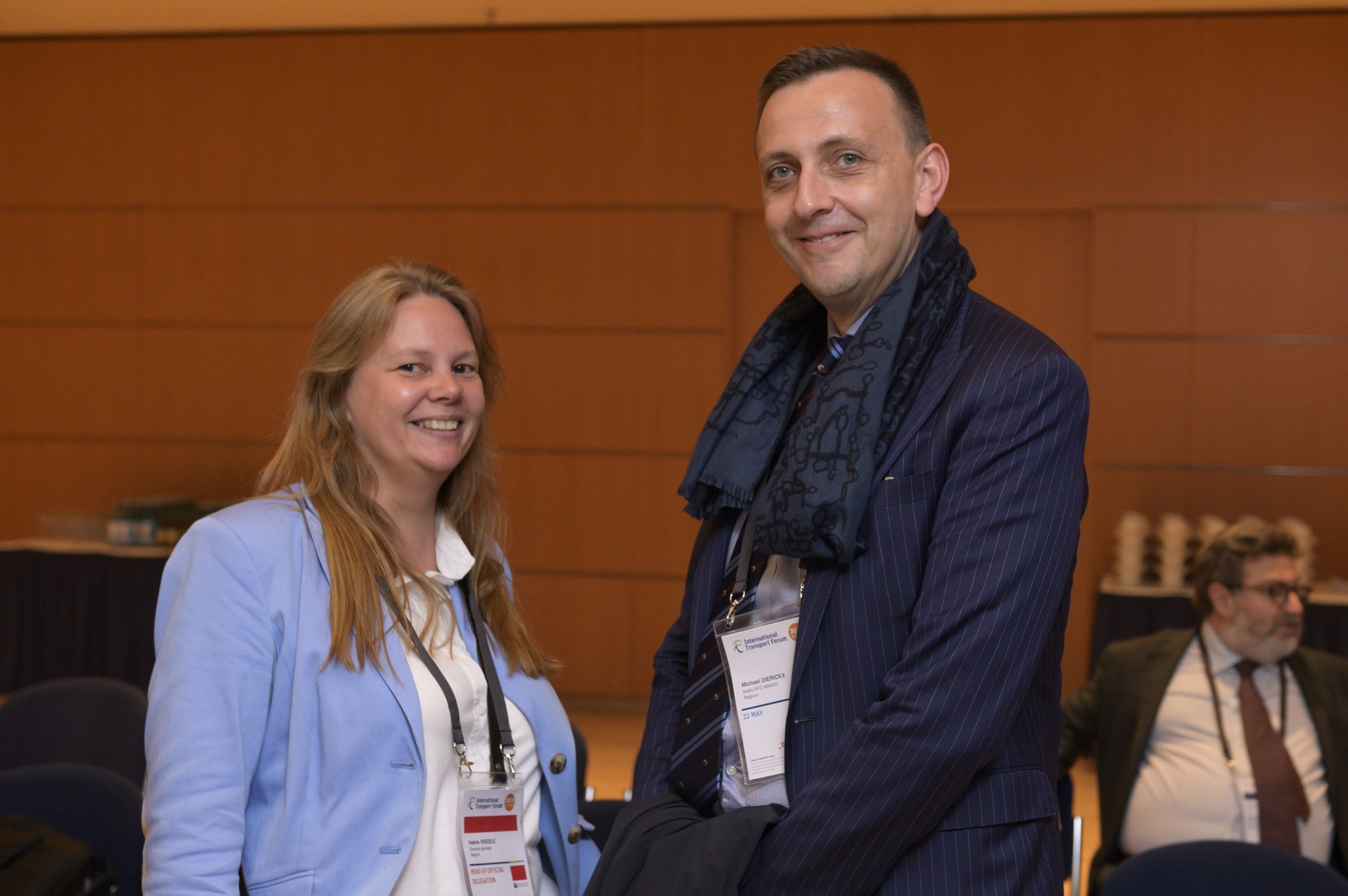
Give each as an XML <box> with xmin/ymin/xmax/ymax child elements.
<box><xmin>1233</xmin><ymin>580</ymin><xmax>1315</xmax><ymax>605</ymax></box>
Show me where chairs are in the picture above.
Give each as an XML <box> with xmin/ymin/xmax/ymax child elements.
<box><xmin>1</xmin><ymin>680</ymin><xmax>147</xmax><ymax>895</ymax></box>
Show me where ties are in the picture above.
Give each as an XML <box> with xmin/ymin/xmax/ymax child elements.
<box><xmin>1230</xmin><ymin>661</ymin><xmax>1310</xmax><ymax>864</ymax></box>
<box><xmin>667</xmin><ymin>336</ymin><xmax>851</xmax><ymax>823</ymax></box>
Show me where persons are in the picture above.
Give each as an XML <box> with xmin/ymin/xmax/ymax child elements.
<box><xmin>1061</xmin><ymin>517</ymin><xmax>1348</xmax><ymax>895</ymax></box>
<box><xmin>141</xmin><ymin>262</ymin><xmax>607</xmax><ymax>896</ymax></box>
<box><xmin>625</xmin><ymin>43</ymin><xmax>1089</xmax><ymax>896</ymax></box>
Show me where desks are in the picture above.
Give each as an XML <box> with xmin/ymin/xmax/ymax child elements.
<box><xmin>1</xmin><ymin>536</ymin><xmax>175</xmax><ymax>694</ymax></box>
